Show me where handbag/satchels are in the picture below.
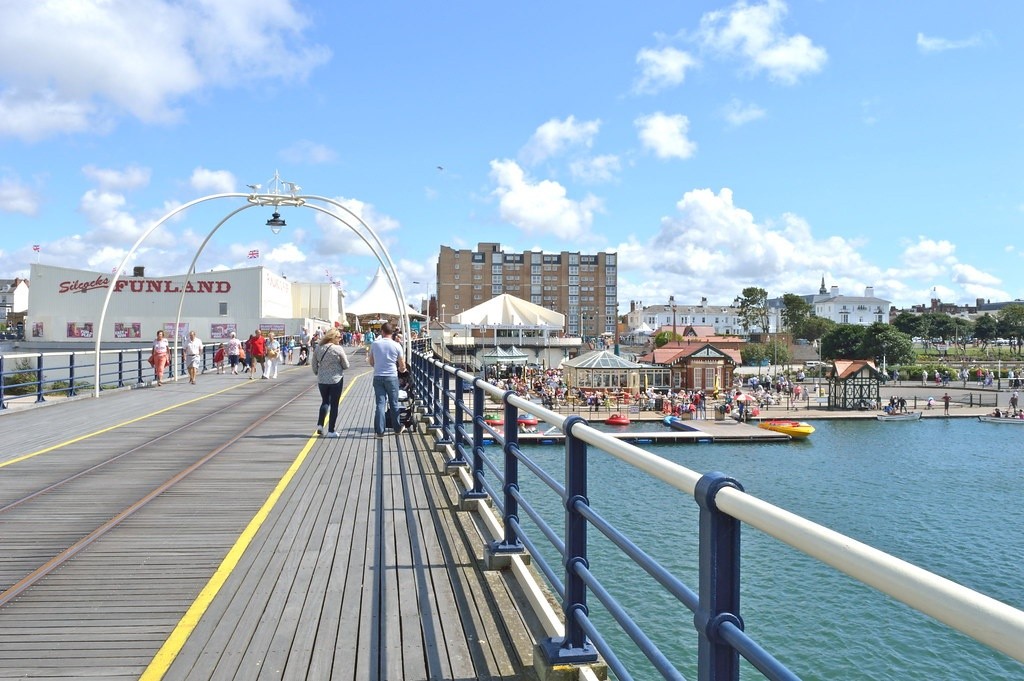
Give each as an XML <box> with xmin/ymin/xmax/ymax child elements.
<box><xmin>165</xmin><ymin>357</ymin><xmax>169</xmax><ymax>367</ymax></box>
<box><xmin>267</xmin><ymin>349</ymin><xmax>276</xmax><ymax>357</ymax></box>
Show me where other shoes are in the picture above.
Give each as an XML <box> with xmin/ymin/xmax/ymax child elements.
<box><xmin>216</xmin><ymin>366</ymin><xmax>277</xmax><ymax>381</ymax></box>
<box><xmin>158</xmin><ymin>380</ymin><xmax>162</xmax><ymax>386</ymax></box>
<box><xmin>189</xmin><ymin>377</ymin><xmax>195</xmax><ymax>384</ymax></box>
<box><xmin>376</xmin><ymin>433</ymin><xmax>384</xmax><ymax>439</ymax></box>
<box><xmin>395</xmin><ymin>425</ymin><xmax>405</xmax><ymax>435</ymax></box>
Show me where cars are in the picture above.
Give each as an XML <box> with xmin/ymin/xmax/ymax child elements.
<box><xmin>462</xmin><ymin>379</ymin><xmax>474</xmax><ymax>392</ymax></box>
<box><xmin>991</xmin><ymin>337</ymin><xmax>1004</xmax><ymax>343</ymax></box>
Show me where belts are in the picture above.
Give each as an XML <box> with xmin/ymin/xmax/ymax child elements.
<box><xmin>188</xmin><ymin>354</ymin><xmax>195</xmax><ymax>356</ymax></box>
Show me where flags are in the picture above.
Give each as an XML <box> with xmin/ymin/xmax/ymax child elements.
<box><xmin>33</xmin><ymin>245</ymin><xmax>40</xmax><ymax>252</ymax></box>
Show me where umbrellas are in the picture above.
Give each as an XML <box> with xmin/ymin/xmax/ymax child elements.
<box><xmin>734</xmin><ymin>394</ymin><xmax>756</xmax><ymax>411</ymax></box>
<box><xmin>633</xmin><ymin>322</ymin><xmax>653</xmax><ymax>342</ymax></box>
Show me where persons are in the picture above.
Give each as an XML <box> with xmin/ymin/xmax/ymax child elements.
<box><xmin>392</xmin><ymin>327</ymin><xmax>426</xmax><ymax>343</ymax></box>
<box><xmin>151</xmin><ymin>330</ymin><xmax>170</xmax><ymax>386</ymax></box>
<box><xmin>398</xmin><ymin>377</ymin><xmax>404</xmax><ymax>388</ymax></box>
<box><xmin>283</xmin><ymin>336</ymin><xmax>296</xmax><ymax>365</ymax></box>
<box><xmin>340</xmin><ymin>329</ymin><xmax>381</xmax><ymax>355</ymax></box>
<box><xmin>250</xmin><ymin>329</ymin><xmax>267</xmax><ymax>379</ymax></box>
<box><xmin>311</xmin><ymin>327</ymin><xmax>324</xmax><ymax>352</ymax></box>
<box><xmin>228</xmin><ymin>332</ymin><xmax>241</xmax><ymax>375</ymax></box>
<box><xmin>564</xmin><ymin>331</ymin><xmax>631</xmax><ymax>349</ymax></box>
<box><xmin>299</xmin><ymin>328</ymin><xmax>310</xmax><ymax>354</ymax></box>
<box><xmin>239</xmin><ymin>346</ymin><xmax>249</xmax><ymax>373</ymax></box>
<box><xmin>262</xmin><ymin>333</ymin><xmax>280</xmax><ymax>379</ymax></box>
<box><xmin>888</xmin><ymin>396</ymin><xmax>907</xmax><ymax>414</ymax></box>
<box><xmin>370</xmin><ymin>323</ymin><xmax>409</xmax><ymax>439</ymax></box>
<box><xmin>893</xmin><ymin>370</ymin><xmax>899</xmax><ymax>384</ymax></box>
<box><xmin>488</xmin><ymin>361</ymin><xmax>809</xmax><ymax>424</ymax></box>
<box><xmin>923</xmin><ymin>367</ymin><xmax>1024</xmax><ymax>420</ymax></box>
<box><xmin>216</xmin><ymin>343</ymin><xmax>226</xmax><ymax>374</ymax></box>
<box><xmin>244</xmin><ymin>335</ymin><xmax>256</xmax><ymax>372</ymax></box>
<box><xmin>299</xmin><ymin>351</ymin><xmax>306</xmax><ymax>365</ymax></box>
<box><xmin>311</xmin><ymin>330</ymin><xmax>349</xmax><ymax>437</ymax></box>
<box><xmin>183</xmin><ymin>331</ymin><xmax>203</xmax><ymax>384</ymax></box>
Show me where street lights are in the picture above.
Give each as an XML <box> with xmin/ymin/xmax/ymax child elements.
<box><xmin>766</xmin><ymin>332</ymin><xmax>777</xmax><ymax>389</ymax></box>
<box><xmin>413</xmin><ymin>281</ymin><xmax>428</xmax><ymax>334</ymax></box>
<box><xmin>813</xmin><ymin>338</ymin><xmax>822</xmax><ymax>407</ymax></box>
<box><xmin>442</xmin><ymin>303</ymin><xmax>446</xmax><ymax>322</ymax></box>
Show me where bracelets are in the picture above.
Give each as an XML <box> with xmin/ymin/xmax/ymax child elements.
<box><xmin>401</xmin><ymin>369</ymin><xmax>407</xmax><ymax>373</ymax></box>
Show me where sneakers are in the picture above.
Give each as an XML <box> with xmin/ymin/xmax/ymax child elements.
<box><xmin>317</xmin><ymin>425</ymin><xmax>323</xmax><ymax>435</ymax></box>
<box><xmin>328</xmin><ymin>431</ymin><xmax>339</xmax><ymax>438</ymax></box>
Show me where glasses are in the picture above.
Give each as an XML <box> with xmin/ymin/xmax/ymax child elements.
<box><xmin>335</xmin><ymin>336</ymin><xmax>341</xmax><ymax>339</ymax></box>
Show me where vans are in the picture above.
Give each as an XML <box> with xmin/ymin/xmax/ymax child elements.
<box><xmin>804</xmin><ymin>361</ymin><xmax>826</xmax><ymax>371</ymax></box>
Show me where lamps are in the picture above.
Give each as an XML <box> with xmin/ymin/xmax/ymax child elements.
<box><xmin>266</xmin><ymin>206</ymin><xmax>286</xmax><ymax>232</ymax></box>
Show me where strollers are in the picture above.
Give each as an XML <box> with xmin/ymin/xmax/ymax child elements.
<box><xmin>385</xmin><ymin>360</ymin><xmax>418</xmax><ymax>432</ymax></box>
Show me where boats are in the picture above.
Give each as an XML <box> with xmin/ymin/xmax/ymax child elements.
<box><xmin>978</xmin><ymin>415</ymin><xmax>1024</xmax><ymax>424</ymax></box>
<box><xmin>877</xmin><ymin>411</ymin><xmax>922</xmax><ymax>421</ymax></box>
<box><xmin>759</xmin><ymin>421</ymin><xmax>816</xmax><ymax>439</ymax></box>
<box><xmin>517</xmin><ymin>413</ymin><xmax>538</xmax><ymax>424</ymax></box>
<box><xmin>484</xmin><ymin>413</ymin><xmax>504</xmax><ymax>425</ymax></box>
<box><xmin>605</xmin><ymin>414</ymin><xmax>630</xmax><ymax>424</ymax></box>
